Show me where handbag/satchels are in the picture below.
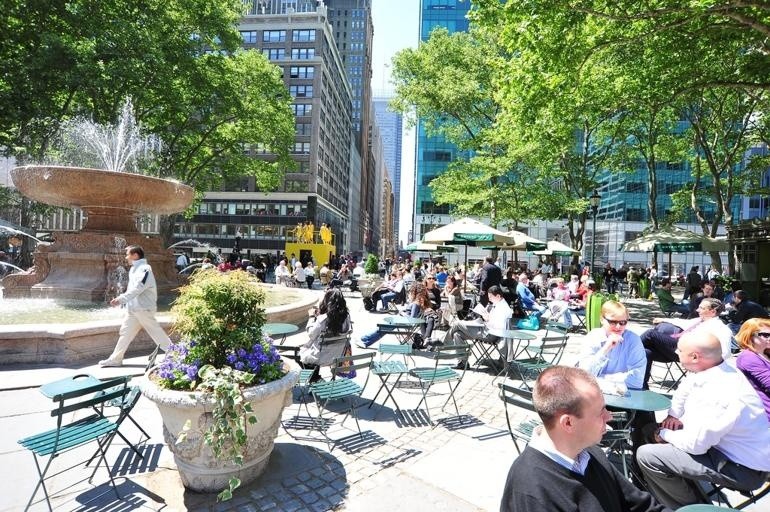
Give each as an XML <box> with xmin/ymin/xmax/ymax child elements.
<box><xmin>363</xmin><ymin>297</ymin><xmax>374</xmax><ymax>310</ymax></box>
<box><xmin>332</xmin><ymin>342</ymin><xmax>356</xmax><ymax>379</ymax></box>
<box><xmin>412</xmin><ymin>334</ymin><xmax>427</xmax><ymax>350</ymax></box>
<box><xmin>516</xmin><ymin>317</ymin><xmax>539</xmax><ymax>330</ymax></box>
<box><xmin>654</xmin><ymin>322</ymin><xmax>684</xmax><ymax>336</ymax></box>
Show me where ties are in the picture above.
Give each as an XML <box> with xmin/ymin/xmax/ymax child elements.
<box><xmin>669</xmin><ymin>318</ymin><xmax>705</xmax><ymax>340</ymax></box>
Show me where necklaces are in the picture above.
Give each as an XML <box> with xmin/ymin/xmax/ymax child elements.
<box><xmin>757</xmin><ymin>353</ymin><xmax>769</xmax><ymax>364</ymax></box>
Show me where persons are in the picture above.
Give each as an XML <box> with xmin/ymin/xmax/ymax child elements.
<box><xmin>99</xmin><ymin>245</ymin><xmax>175</xmax><ymax>367</ymax></box>
<box><xmin>499</xmin><ymin>363</ymin><xmax>671</xmax><ymax>511</ymax></box>
<box><xmin>573</xmin><ymin>299</ymin><xmax>658</xmax><ymax>492</ymax></box>
<box><xmin>636</xmin><ymin>332</ymin><xmax>770</xmax><ymax>511</ymax></box>
<box><xmin>734</xmin><ymin>318</ymin><xmax>770</xmax><ymax>421</ymax></box>
<box><xmin>175</xmin><ymin>251</ymin><xmax>764</xmax><ymax>394</ymax></box>
<box><xmin>289</xmin><ymin>222</ymin><xmax>332</xmax><ymax>244</ymax></box>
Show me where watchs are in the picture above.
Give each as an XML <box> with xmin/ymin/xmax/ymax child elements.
<box><xmin>656</xmin><ymin>427</ymin><xmax>663</xmax><ymax>440</ymax></box>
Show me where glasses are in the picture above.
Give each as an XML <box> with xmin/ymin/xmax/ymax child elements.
<box><xmin>603</xmin><ymin>315</ymin><xmax>627</xmax><ymax>325</ymax></box>
<box><xmin>752</xmin><ymin>331</ymin><xmax>769</xmax><ymax>338</ymax></box>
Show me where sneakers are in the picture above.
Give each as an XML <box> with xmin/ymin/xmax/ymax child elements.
<box><xmin>379</xmin><ymin>308</ymin><xmax>389</xmax><ymax>312</ymax></box>
<box><xmin>356</xmin><ymin>342</ymin><xmax>366</xmax><ymax>348</ymax></box>
<box><xmin>99</xmin><ymin>359</ymin><xmax>122</xmax><ymax>367</ymax></box>
<box><xmin>308</xmin><ymin>379</ymin><xmax>327</xmax><ymax>395</ymax></box>
<box><xmin>423</xmin><ymin>338</ymin><xmax>430</xmax><ymax>348</ymax></box>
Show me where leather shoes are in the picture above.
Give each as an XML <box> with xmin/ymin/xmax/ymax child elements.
<box><xmin>452</xmin><ymin>361</ymin><xmax>470</xmax><ymax>370</ymax></box>
<box><xmin>428</xmin><ymin>339</ymin><xmax>443</xmax><ymax>346</ymax></box>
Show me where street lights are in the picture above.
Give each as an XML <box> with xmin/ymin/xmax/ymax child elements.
<box><xmin>232</xmin><ymin>231</ymin><xmax>242</xmax><ymax>255</ymax></box>
<box><xmin>587</xmin><ymin>187</ymin><xmax>602</xmax><ymax>277</ymax></box>
